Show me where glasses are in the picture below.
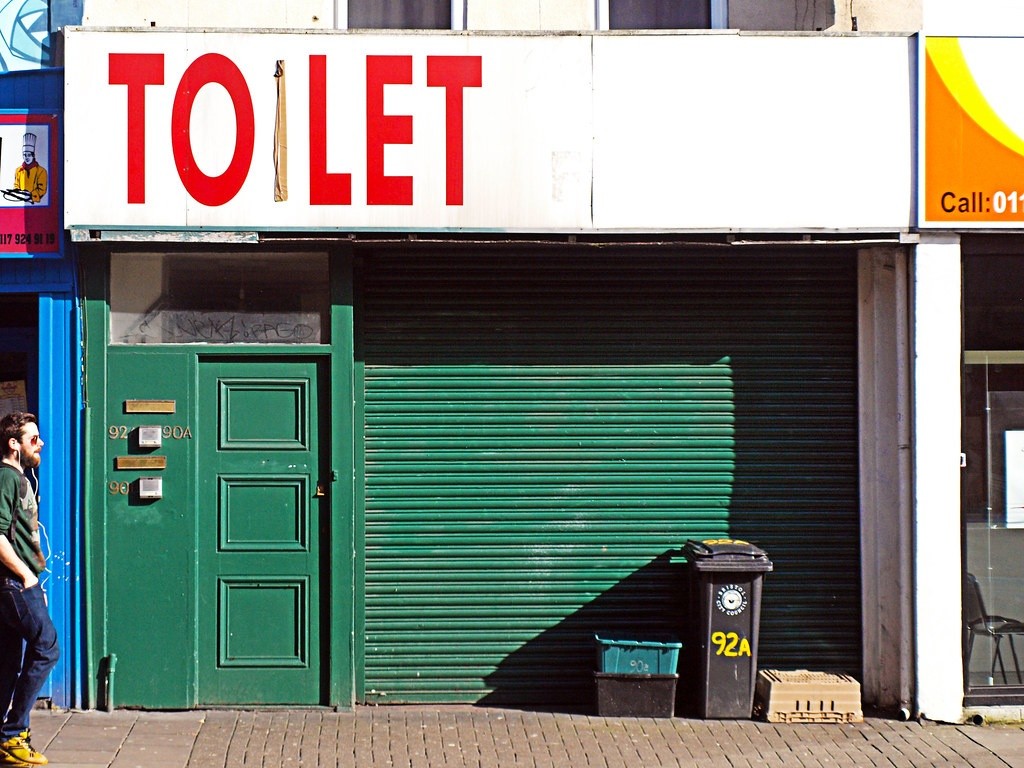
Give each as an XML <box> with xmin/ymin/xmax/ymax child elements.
<box><xmin>16</xmin><ymin>435</ymin><xmax>39</xmax><ymax>446</ymax></box>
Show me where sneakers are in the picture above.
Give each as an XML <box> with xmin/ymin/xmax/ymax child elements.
<box><xmin>0</xmin><ymin>732</ymin><xmax>48</xmax><ymax>766</ymax></box>
<box><xmin>0</xmin><ymin>748</ymin><xmax>30</xmax><ymax>767</ymax></box>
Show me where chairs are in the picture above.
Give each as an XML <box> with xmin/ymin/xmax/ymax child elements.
<box><xmin>966</xmin><ymin>572</ymin><xmax>1024</xmax><ymax>687</ymax></box>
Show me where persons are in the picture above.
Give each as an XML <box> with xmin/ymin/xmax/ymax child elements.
<box><xmin>0</xmin><ymin>412</ymin><xmax>59</xmax><ymax>768</ymax></box>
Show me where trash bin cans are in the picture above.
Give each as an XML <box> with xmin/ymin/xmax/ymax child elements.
<box><xmin>673</xmin><ymin>539</ymin><xmax>774</xmax><ymax>719</ymax></box>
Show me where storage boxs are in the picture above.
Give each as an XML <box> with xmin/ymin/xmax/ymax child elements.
<box><xmin>756</xmin><ymin>668</ymin><xmax>867</xmax><ymax>723</ymax></box>
<box><xmin>593</xmin><ymin>631</ymin><xmax>681</xmax><ymax>718</ymax></box>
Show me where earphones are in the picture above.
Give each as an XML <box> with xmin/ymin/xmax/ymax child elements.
<box><xmin>11</xmin><ymin>441</ymin><xmax>15</xmax><ymax>446</ymax></box>
